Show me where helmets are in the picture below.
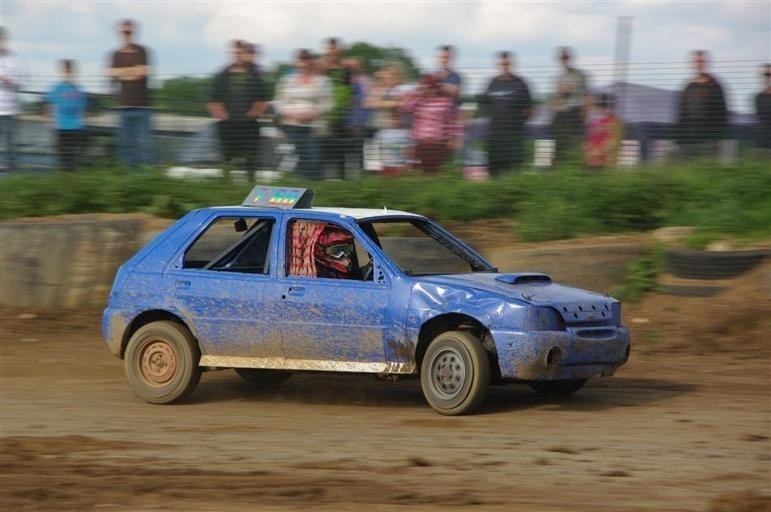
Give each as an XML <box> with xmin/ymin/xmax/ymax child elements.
<box><xmin>314</xmin><ymin>227</ymin><xmax>356</xmax><ymax>274</ymax></box>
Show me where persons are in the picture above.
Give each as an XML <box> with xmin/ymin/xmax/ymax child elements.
<box><xmin>45</xmin><ymin>60</ymin><xmax>88</xmax><ymax>173</ymax></box>
<box><xmin>318</xmin><ymin>37</ymin><xmax>354</xmax><ymax>181</ymax></box>
<box><xmin>1</xmin><ymin>26</ymin><xmax>27</xmax><ymax>172</ymax></box>
<box><xmin>272</xmin><ymin>46</ymin><xmax>337</xmax><ymax>181</ymax></box>
<box><xmin>104</xmin><ymin>17</ymin><xmax>158</xmax><ymax>168</ymax></box>
<box><xmin>207</xmin><ymin>39</ymin><xmax>267</xmax><ymax>183</ymax></box>
<box><xmin>312</xmin><ymin>226</ymin><xmax>375</xmax><ymax>282</ymax></box>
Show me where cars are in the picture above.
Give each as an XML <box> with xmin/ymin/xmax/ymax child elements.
<box><xmin>102</xmin><ymin>184</ymin><xmax>630</xmax><ymax>416</ymax></box>
